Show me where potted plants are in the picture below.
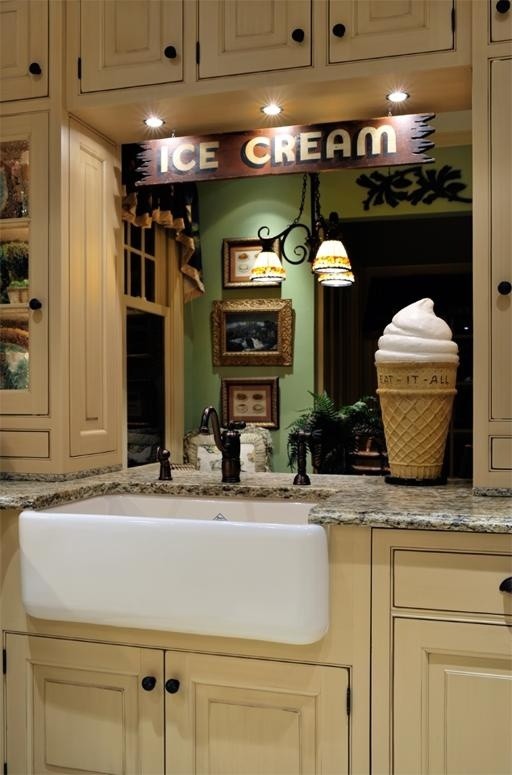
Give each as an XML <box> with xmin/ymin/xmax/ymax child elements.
<box><xmin>0</xmin><ymin>239</ymin><xmax>30</xmax><ymax>304</ymax></box>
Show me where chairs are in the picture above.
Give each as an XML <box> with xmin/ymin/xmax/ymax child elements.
<box><xmin>182</xmin><ymin>423</ymin><xmax>273</xmax><ymax>476</ymax></box>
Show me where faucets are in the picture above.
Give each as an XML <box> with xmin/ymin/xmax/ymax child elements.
<box><xmin>154</xmin><ymin>444</ymin><xmax>166</xmax><ymax>466</ymax></box>
<box><xmin>199</xmin><ymin>404</ymin><xmax>227</xmax><ymax>457</ymax></box>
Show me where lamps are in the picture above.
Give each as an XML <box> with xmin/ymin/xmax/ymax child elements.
<box><xmin>249</xmin><ymin>170</ymin><xmax>355</xmax><ymax>290</ymax></box>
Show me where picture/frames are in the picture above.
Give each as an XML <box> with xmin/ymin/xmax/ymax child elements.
<box><xmin>220</xmin><ymin>236</ymin><xmax>282</xmax><ymax>289</ymax></box>
<box><xmin>220</xmin><ymin>377</ymin><xmax>280</xmax><ymax>430</ymax></box>
<box><xmin>209</xmin><ymin>297</ymin><xmax>295</xmax><ymax>368</ymax></box>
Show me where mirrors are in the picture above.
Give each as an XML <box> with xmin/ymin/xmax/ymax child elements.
<box><xmin>119</xmin><ymin>99</ymin><xmax>477</xmax><ymax>481</ymax></box>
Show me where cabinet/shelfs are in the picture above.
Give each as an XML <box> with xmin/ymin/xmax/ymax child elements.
<box><xmin>73</xmin><ymin>2</ymin><xmax>191</xmax><ymax>96</ymax></box>
<box><xmin>367</xmin><ymin>532</ymin><xmax>510</xmax><ymax>774</ymax></box>
<box><xmin>0</xmin><ymin>624</ymin><xmax>364</xmax><ymax>775</ymax></box>
<box><xmin>473</xmin><ymin>45</ymin><xmax>512</xmax><ymax>486</ymax></box>
<box><xmin>0</xmin><ymin>108</ymin><xmax>123</xmax><ymax>479</ymax></box>
<box><xmin>1</xmin><ymin>2</ymin><xmax>64</xmax><ymax>103</ymax></box>
<box><xmin>187</xmin><ymin>2</ymin><xmax>472</xmax><ymax>84</ymax></box>
<box><xmin>480</xmin><ymin>2</ymin><xmax>512</xmax><ymax>51</ymax></box>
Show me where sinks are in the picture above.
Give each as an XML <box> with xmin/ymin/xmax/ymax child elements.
<box><xmin>18</xmin><ymin>493</ymin><xmax>332</xmax><ymax>649</ymax></box>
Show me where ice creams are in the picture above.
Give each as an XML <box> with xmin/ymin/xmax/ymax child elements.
<box><xmin>374</xmin><ymin>295</ymin><xmax>460</xmax><ymax>480</ymax></box>
